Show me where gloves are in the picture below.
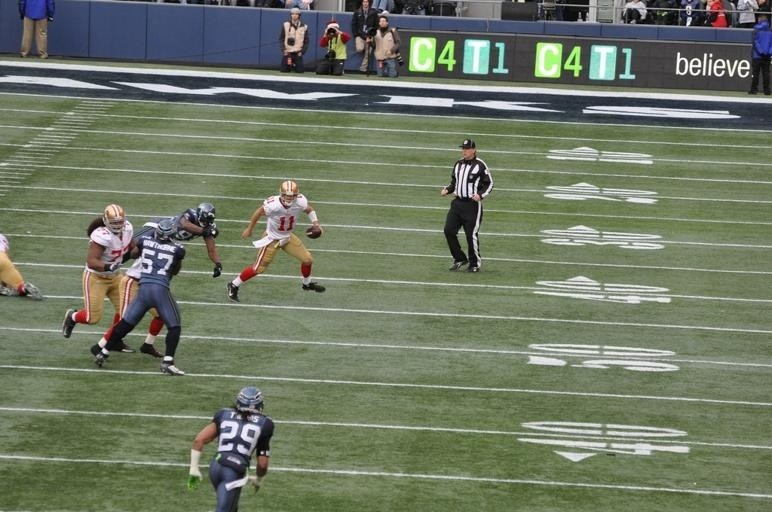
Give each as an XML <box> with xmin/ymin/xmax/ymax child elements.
<box><xmin>187</xmin><ymin>449</ymin><xmax>203</xmax><ymax>492</ymax></box>
<box><xmin>249</xmin><ymin>475</ymin><xmax>265</xmax><ymax>494</ymax></box>
<box><xmin>109</xmin><ymin>262</ymin><xmax>120</xmax><ymax>271</ymax></box>
<box><xmin>213</xmin><ymin>263</ymin><xmax>222</xmax><ymax>277</ymax></box>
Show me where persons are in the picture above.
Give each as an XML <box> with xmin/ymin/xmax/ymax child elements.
<box><xmin>122</xmin><ymin>202</ymin><xmax>222</xmax><ymax>277</ymax></box>
<box><xmin>0</xmin><ymin>229</ymin><xmax>43</xmax><ymax>300</ymax></box>
<box><xmin>225</xmin><ymin>181</ymin><xmax>326</xmax><ymax>301</ymax></box>
<box><xmin>353</xmin><ymin>0</ymin><xmax>378</xmax><ymax>73</ymax></box>
<box><xmin>95</xmin><ymin>220</ymin><xmax>187</xmax><ymax>375</ymax></box>
<box><xmin>188</xmin><ymin>385</ymin><xmax>274</xmax><ymax>511</ymax></box>
<box><xmin>372</xmin><ymin>15</ymin><xmax>404</xmax><ymax>78</ymax></box>
<box><xmin>315</xmin><ymin>21</ymin><xmax>351</xmax><ymax>76</ymax></box>
<box><xmin>18</xmin><ymin>1</ymin><xmax>55</xmax><ymax>59</ymax></box>
<box><xmin>92</xmin><ymin>255</ymin><xmax>163</xmax><ymax>359</ymax></box>
<box><xmin>440</xmin><ymin>138</ymin><xmax>493</xmax><ymax>272</ymax></box>
<box><xmin>279</xmin><ymin>7</ymin><xmax>309</xmax><ymax>74</ymax></box>
<box><xmin>750</xmin><ymin>15</ymin><xmax>772</xmax><ymax>96</ymax></box>
<box><xmin>63</xmin><ymin>205</ymin><xmax>140</xmax><ymax>354</ymax></box>
<box><xmin>621</xmin><ymin>0</ymin><xmax>771</xmax><ymax>31</ymax></box>
<box><xmin>370</xmin><ymin>0</ymin><xmax>461</xmax><ymax>18</ymax></box>
<box><xmin>281</xmin><ymin>0</ymin><xmax>315</xmax><ymax>10</ymax></box>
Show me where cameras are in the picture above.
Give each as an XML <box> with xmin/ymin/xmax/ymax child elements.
<box><xmin>325</xmin><ymin>50</ymin><xmax>335</xmax><ymax>61</ymax></box>
<box><xmin>328</xmin><ymin>29</ymin><xmax>336</xmax><ymax>38</ymax></box>
<box><xmin>287</xmin><ymin>38</ymin><xmax>296</xmax><ymax>46</ymax></box>
<box><xmin>366</xmin><ymin>27</ymin><xmax>377</xmax><ymax>40</ymax></box>
<box><xmin>396</xmin><ymin>49</ymin><xmax>404</xmax><ymax>67</ymax></box>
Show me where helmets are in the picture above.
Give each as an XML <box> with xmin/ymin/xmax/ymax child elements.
<box><xmin>196</xmin><ymin>203</ymin><xmax>216</xmax><ymax>229</ymax></box>
<box><xmin>102</xmin><ymin>203</ymin><xmax>126</xmax><ymax>233</ymax></box>
<box><xmin>235</xmin><ymin>387</ymin><xmax>265</xmax><ymax>415</ymax></box>
<box><xmin>153</xmin><ymin>219</ymin><xmax>179</xmax><ymax>243</ymax></box>
<box><xmin>279</xmin><ymin>181</ymin><xmax>299</xmax><ymax>207</ymax></box>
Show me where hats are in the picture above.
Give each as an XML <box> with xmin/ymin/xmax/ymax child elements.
<box><xmin>291</xmin><ymin>8</ymin><xmax>300</xmax><ymax>14</ymax></box>
<box><xmin>459</xmin><ymin>139</ymin><xmax>475</xmax><ymax>148</ymax></box>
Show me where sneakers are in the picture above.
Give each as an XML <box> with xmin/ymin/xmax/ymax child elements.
<box><xmin>0</xmin><ymin>285</ymin><xmax>19</xmax><ymax>296</ymax></box>
<box><xmin>62</xmin><ymin>309</ymin><xmax>77</xmax><ymax>339</ymax></box>
<box><xmin>90</xmin><ymin>339</ymin><xmax>135</xmax><ymax>366</ymax></box>
<box><xmin>465</xmin><ymin>267</ymin><xmax>479</xmax><ymax>273</ymax></box>
<box><xmin>302</xmin><ymin>282</ymin><xmax>325</xmax><ymax>292</ymax></box>
<box><xmin>449</xmin><ymin>260</ymin><xmax>468</xmax><ymax>272</ymax></box>
<box><xmin>226</xmin><ymin>281</ymin><xmax>240</xmax><ymax>303</ymax></box>
<box><xmin>24</xmin><ymin>282</ymin><xmax>42</xmax><ymax>300</ymax></box>
<box><xmin>140</xmin><ymin>343</ymin><xmax>165</xmax><ymax>357</ymax></box>
<box><xmin>159</xmin><ymin>362</ymin><xmax>184</xmax><ymax>375</ymax></box>
